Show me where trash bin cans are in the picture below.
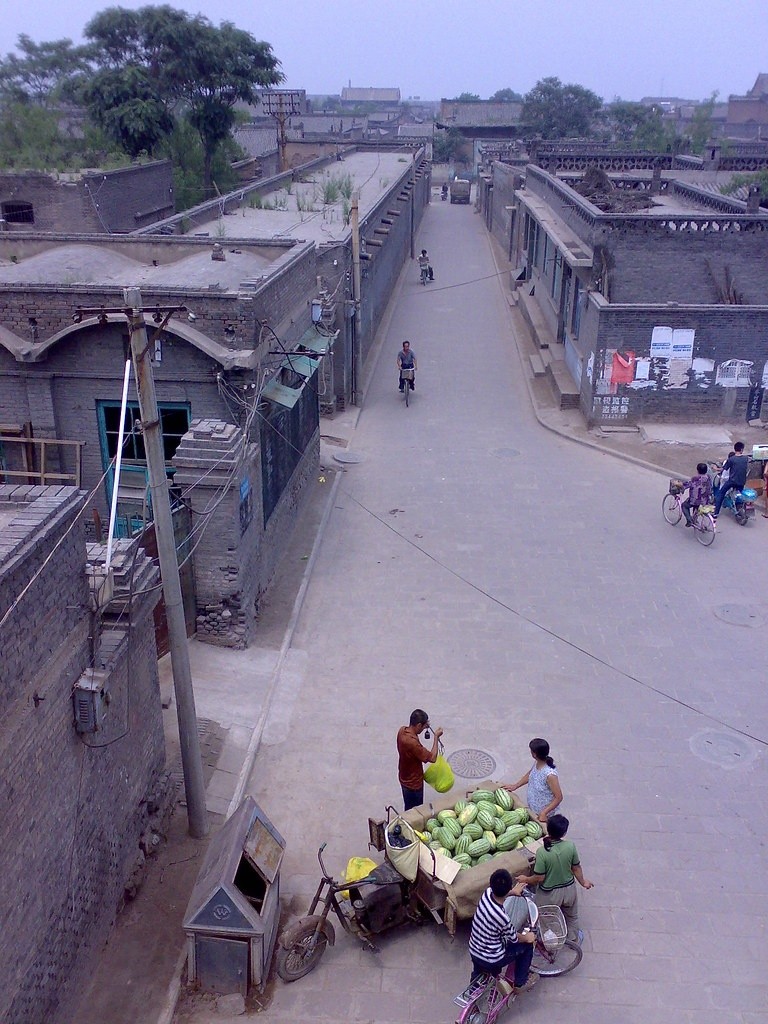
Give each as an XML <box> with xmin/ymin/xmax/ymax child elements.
<box><xmin>181</xmin><ymin>795</ymin><xmax>288</xmax><ymax>999</ymax></box>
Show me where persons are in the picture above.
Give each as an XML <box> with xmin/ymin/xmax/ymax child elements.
<box><xmin>469</xmin><ymin>869</ymin><xmax>540</xmax><ymax>1001</ymax></box>
<box><xmin>396</xmin><ymin>341</ymin><xmax>418</xmax><ymax>393</ymax></box>
<box><xmin>416</xmin><ymin>250</ymin><xmax>436</xmax><ymax>281</ymax></box>
<box><xmin>500</xmin><ymin>738</ymin><xmax>564</xmax><ymax>822</ymax></box>
<box><xmin>515</xmin><ymin>814</ymin><xmax>595</xmax><ymax>949</ymax></box>
<box><xmin>674</xmin><ymin>463</ymin><xmax>712</xmax><ymax>527</ymax></box>
<box><xmin>442</xmin><ymin>182</ymin><xmax>448</xmax><ymax>194</ymax></box>
<box><xmin>396</xmin><ymin>709</ymin><xmax>443</xmax><ymax>811</ymax></box>
<box><xmin>711</xmin><ymin>442</ymin><xmax>748</xmax><ymax>519</ymax></box>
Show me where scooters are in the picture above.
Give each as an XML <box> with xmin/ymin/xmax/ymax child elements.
<box><xmin>707</xmin><ymin>461</ymin><xmax>759</xmax><ymax>526</ymax></box>
<box><xmin>441</xmin><ymin>191</ymin><xmax>447</xmax><ymax>201</ymax></box>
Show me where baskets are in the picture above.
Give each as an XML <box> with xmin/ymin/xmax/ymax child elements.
<box><xmin>669</xmin><ymin>478</ymin><xmax>683</xmax><ymax>494</ymax></box>
<box><xmin>420</xmin><ymin>263</ymin><xmax>428</xmax><ymax>270</ymax></box>
<box><xmin>538</xmin><ymin>905</ymin><xmax>567</xmax><ymax>952</ymax></box>
<box><xmin>402</xmin><ymin>370</ymin><xmax>414</xmax><ymax>379</ymax></box>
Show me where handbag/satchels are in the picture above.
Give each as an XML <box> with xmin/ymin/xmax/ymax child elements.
<box><xmin>386</xmin><ymin>815</ymin><xmax>420</xmax><ymax>883</ymax></box>
<box><xmin>340</xmin><ymin>857</ymin><xmax>379</xmax><ymax>900</ymax></box>
<box><xmin>423</xmin><ymin>754</ymin><xmax>454</xmax><ymax>793</ymax></box>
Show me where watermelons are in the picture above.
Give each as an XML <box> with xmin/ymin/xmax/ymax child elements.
<box><xmin>424</xmin><ymin>768</ymin><xmax>455</xmax><ymax>792</ymax></box>
<box><xmin>421</xmin><ymin>788</ymin><xmax>543</xmax><ymax>870</ymax></box>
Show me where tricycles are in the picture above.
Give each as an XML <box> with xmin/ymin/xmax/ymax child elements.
<box><xmin>275</xmin><ymin>779</ymin><xmax>551</xmax><ymax>982</ymax></box>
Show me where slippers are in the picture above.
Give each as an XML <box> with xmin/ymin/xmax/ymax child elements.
<box><xmin>570</xmin><ymin>928</ymin><xmax>584</xmax><ymax>950</ymax></box>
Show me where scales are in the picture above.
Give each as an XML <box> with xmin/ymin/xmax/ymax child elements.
<box><xmin>425</xmin><ymin>721</ymin><xmax>445</xmax><ymax>756</ymax></box>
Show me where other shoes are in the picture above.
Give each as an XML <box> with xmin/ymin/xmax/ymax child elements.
<box><xmin>711</xmin><ymin>515</ymin><xmax>717</xmax><ymax>519</ymax></box>
<box><xmin>421</xmin><ymin>279</ymin><xmax>423</xmax><ymax>280</ymax></box>
<box><xmin>400</xmin><ymin>391</ymin><xmax>404</xmax><ymax>393</ymax></box>
<box><xmin>410</xmin><ymin>386</ymin><xmax>414</xmax><ymax>390</ymax></box>
<box><xmin>685</xmin><ymin>519</ymin><xmax>693</xmax><ymax>527</ymax></box>
<box><xmin>430</xmin><ymin>279</ymin><xmax>434</xmax><ymax>280</ymax></box>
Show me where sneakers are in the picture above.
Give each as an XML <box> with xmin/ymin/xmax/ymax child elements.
<box><xmin>513</xmin><ymin>973</ymin><xmax>540</xmax><ymax>994</ymax></box>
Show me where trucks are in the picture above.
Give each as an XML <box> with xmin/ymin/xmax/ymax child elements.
<box><xmin>450</xmin><ymin>179</ymin><xmax>471</xmax><ymax>204</ymax></box>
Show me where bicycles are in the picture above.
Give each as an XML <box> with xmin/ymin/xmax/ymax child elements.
<box><xmin>454</xmin><ymin>884</ymin><xmax>583</xmax><ymax>1024</ymax></box>
<box><xmin>399</xmin><ymin>367</ymin><xmax>416</xmax><ymax>407</ymax></box>
<box><xmin>661</xmin><ymin>478</ymin><xmax>717</xmax><ymax>546</ymax></box>
<box><xmin>420</xmin><ymin>262</ymin><xmax>429</xmax><ymax>286</ymax></box>
